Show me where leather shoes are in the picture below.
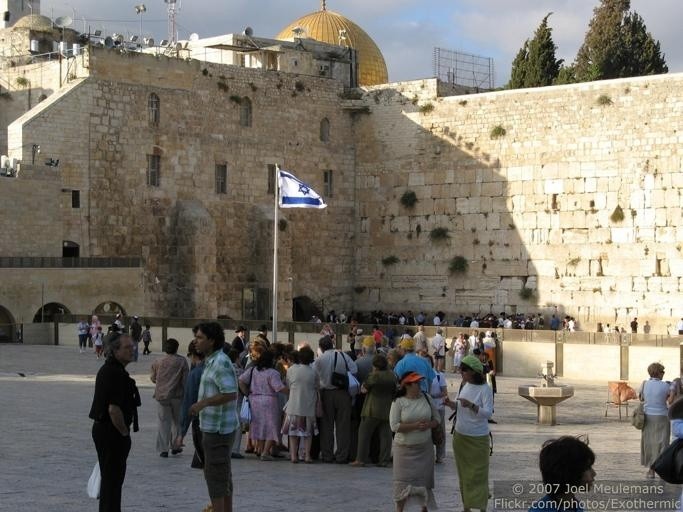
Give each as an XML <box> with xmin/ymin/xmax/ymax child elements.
<box><xmin>644</xmin><ymin>473</ymin><xmax>656</xmax><ymax>479</ymax></box>
<box><xmin>231</xmin><ymin>447</ymin><xmax>387</xmax><ymax>467</ymax></box>
<box><xmin>171</xmin><ymin>448</ymin><xmax>184</xmax><ymax>455</ymax></box>
<box><xmin>159</xmin><ymin>453</ymin><xmax>169</xmax><ymax>458</ymax></box>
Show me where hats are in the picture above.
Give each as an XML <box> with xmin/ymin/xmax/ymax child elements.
<box><xmin>235</xmin><ymin>326</ymin><xmax>247</xmax><ymax>334</ymax></box>
<box><xmin>399</xmin><ymin>371</ymin><xmax>426</xmax><ymax>384</ymax></box>
<box><xmin>399</xmin><ymin>338</ymin><xmax>416</xmax><ymax>350</ymax></box>
<box><xmin>361</xmin><ymin>336</ymin><xmax>376</xmax><ymax>347</ymax></box>
<box><xmin>461</xmin><ymin>355</ymin><xmax>484</xmax><ymax>375</ymax></box>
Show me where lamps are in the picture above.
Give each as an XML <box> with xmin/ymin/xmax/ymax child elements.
<box><xmin>93</xmin><ymin>30</ymin><xmax>190</xmax><ymax>52</ymax></box>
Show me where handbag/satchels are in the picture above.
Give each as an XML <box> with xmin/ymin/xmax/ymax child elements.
<box><xmin>629</xmin><ymin>404</ymin><xmax>645</xmax><ymax>431</ymax></box>
<box><xmin>432</xmin><ymin>423</ymin><xmax>444</xmax><ymax>448</ymax></box>
<box><xmin>316</xmin><ymin>400</ymin><xmax>325</xmax><ymax>419</ymax></box>
<box><xmin>331</xmin><ymin>371</ymin><xmax>350</xmax><ymax>392</ymax></box>
<box><xmin>240</xmin><ymin>395</ymin><xmax>253</xmax><ymax>425</ymax></box>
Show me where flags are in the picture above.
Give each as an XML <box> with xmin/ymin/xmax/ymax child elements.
<box><xmin>277</xmin><ymin>170</ymin><xmax>328</xmax><ymax>210</ymax></box>
